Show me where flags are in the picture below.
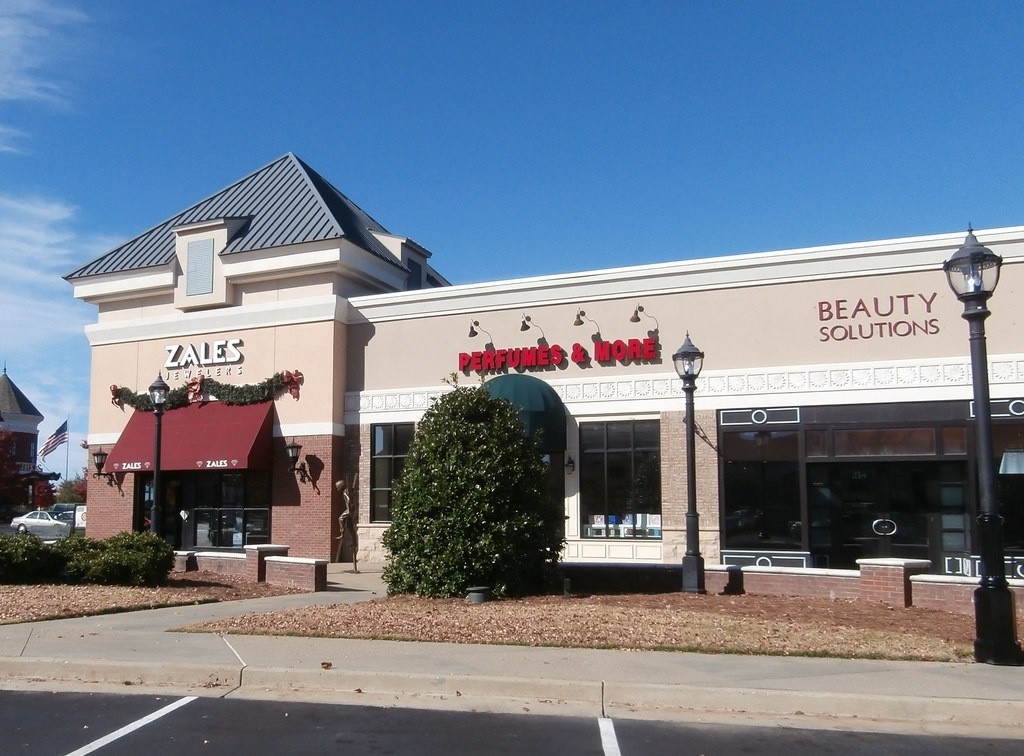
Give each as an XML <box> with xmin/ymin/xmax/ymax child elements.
<box><xmin>38</xmin><ymin>420</ymin><xmax>67</xmax><ymax>461</ymax></box>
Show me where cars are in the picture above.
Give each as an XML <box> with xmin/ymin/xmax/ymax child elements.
<box><xmin>54</xmin><ymin>510</ymin><xmax>75</xmax><ymax>520</ymax></box>
<box><xmin>10</xmin><ymin>510</ymin><xmax>73</xmax><ymax>539</ymax></box>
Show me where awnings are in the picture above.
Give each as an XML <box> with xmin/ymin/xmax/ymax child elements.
<box><xmin>478</xmin><ymin>374</ymin><xmax>567</xmax><ymax>431</ymax></box>
<box><xmin>999</xmin><ymin>451</ymin><xmax>1024</xmax><ymax>474</ymax></box>
<box><xmin>99</xmin><ymin>397</ymin><xmax>273</xmax><ymax>472</ymax></box>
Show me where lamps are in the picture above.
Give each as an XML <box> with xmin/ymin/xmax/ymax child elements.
<box><xmin>284</xmin><ymin>436</ymin><xmax>305</xmax><ymax>483</ymax></box>
<box><xmin>564</xmin><ymin>455</ymin><xmax>574</xmax><ymax>474</ymax></box>
<box><xmin>574</xmin><ymin>306</ymin><xmax>585</xmax><ymax>325</ymax></box>
<box><xmin>630</xmin><ymin>303</ymin><xmax>644</xmax><ymax>321</ymax></box>
<box><xmin>469</xmin><ymin>318</ymin><xmax>479</xmax><ymax>337</ymax></box>
<box><xmin>520</xmin><ymin>313</ymin><xmax>531</xmax><ymax>331</ymax></box>
<box><xmin>92</xmin><ymin>446</ymin><xmax>112</xmax><ymax>486</ymax></box>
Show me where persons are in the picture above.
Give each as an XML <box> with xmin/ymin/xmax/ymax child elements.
<box><xmin>335</xmin><ymin>479</ymin><xmax>358</xmax><ymax>547</ymax></box>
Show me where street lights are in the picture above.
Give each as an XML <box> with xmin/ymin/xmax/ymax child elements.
<box><xmin>671</xmin><ymin>330</ymin><xmax>704</xmax><ymax>594</ymax></box>
<box><xmin>149</xmin><ymin>370</ymin><xmax>171</xmax><ymax>537</ymax></box>
<box><xmin>940</xmin><ymin>223</ymin><xmax>1024</xmax><ymax>666</ymax></box>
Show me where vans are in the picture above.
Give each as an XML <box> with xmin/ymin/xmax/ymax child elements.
<box><xmin>51</xmin><ymin>503</ymin><xmax>85</xmax><ymax>522</ymax></box>
